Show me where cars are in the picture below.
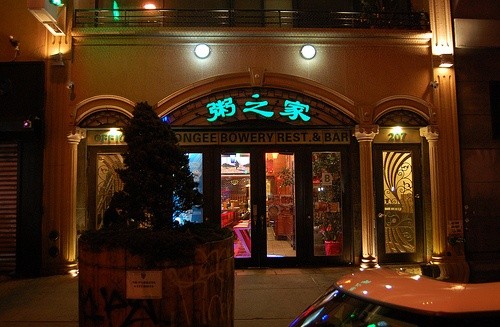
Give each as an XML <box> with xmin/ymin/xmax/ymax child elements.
<box><xmin>286</xmin><ymin>268</ymin><xmax>500</xmax><ymax>327</ymax></box>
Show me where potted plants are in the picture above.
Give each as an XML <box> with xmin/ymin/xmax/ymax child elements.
<box><xmin>77</xmin><ymin>100</ymin><xmax>236</xmax><ymax>327</ymax></box>
